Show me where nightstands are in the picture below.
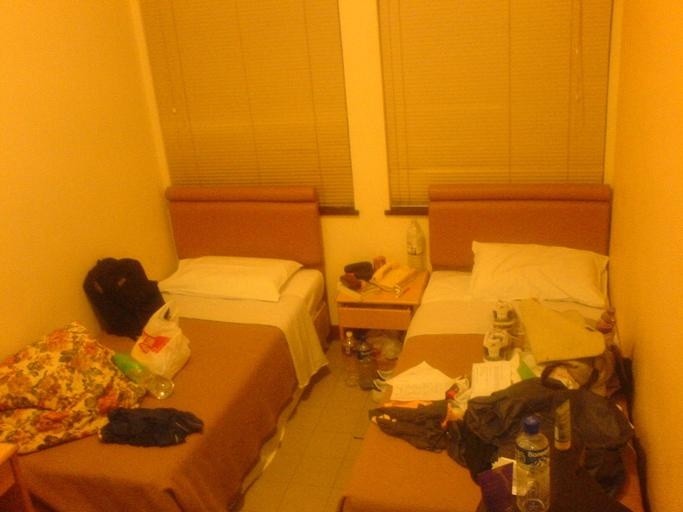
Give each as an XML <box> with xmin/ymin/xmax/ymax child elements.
<box><xmin>336</xmin><ymin>271</ymin><xmax>429</xmax><ymax>352</ymax></box>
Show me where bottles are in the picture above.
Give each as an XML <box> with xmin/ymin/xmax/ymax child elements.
<box><xmin>405</xmin><ymin>218</ymin><xmax>425</xmax><ymax>274</ymax></box>
<box><xmin>112</xmin><ymin>351</ymin><xmax>175</xmax><ymax>400</ymax></box>
<box><xmin>341</xmin><ymin>330</ymin><xmax>360</xmax><ymax>386</ymax></box>
<box><xmin>513</xmin><ymin>414</ymin><xmax>552</xmax><ymax>511</ymax></box>
<box><xmin>354</xmin><ymin>336</ymin><xmax>376</xmax><ymax>390</ymax></box>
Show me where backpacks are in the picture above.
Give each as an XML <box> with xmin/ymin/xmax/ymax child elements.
<box><xmin>83</xmin><ymin>257</ymin><xmax>167</xmax><ymax>336</ymax></box>
<box><xmin>465</xmin><ymin>377</ymin><xmax>633</xmax><ymax>500</ymax></box>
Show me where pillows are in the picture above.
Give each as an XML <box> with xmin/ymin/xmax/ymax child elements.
<box><xmin>156</xmin><ymin>255</ymin><xmax>304</xmax><ymax>302</ymax></box>
<box><xmin>466</xmin><ymin>240</ymin><xmax>609</xmax><ymax>310</ymax></box>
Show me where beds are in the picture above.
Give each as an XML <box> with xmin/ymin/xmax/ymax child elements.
<box><xmin>338</xmin><ymin>182</ymin><xmax>643</xmax><ymax>512</ymax></box>
<box><xmin>9</xmin><ymin>185</ymin><xmax>331</xmax><ymax>512</ymax></box>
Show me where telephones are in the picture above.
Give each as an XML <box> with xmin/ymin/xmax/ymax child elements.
<box><xmin>371</xmin><ymin>261</ymin><xmax>418</xmax><ymax>293</ymax></box>
